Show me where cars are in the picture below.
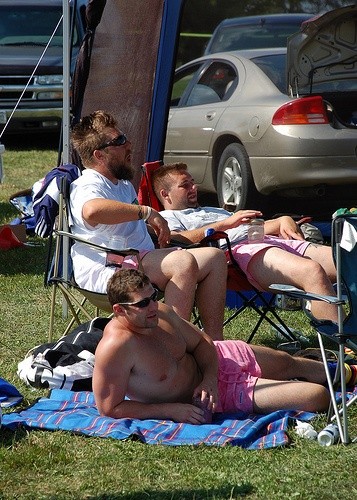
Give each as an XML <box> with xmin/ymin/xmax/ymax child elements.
<box><xmin>200</xmin><ymin>14</ymin><xmax>317</xmax><ymax>56</ymax></box>
<box><xmin>162</xmin><ymin>3</ymin><xmax>357</xmax><ymax>215</ymax></box>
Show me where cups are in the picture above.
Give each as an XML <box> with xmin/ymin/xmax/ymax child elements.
<box><xmin>247</xmin><ymin>218</ymin><xmax>264</xmax><ymax>243</ymax></box>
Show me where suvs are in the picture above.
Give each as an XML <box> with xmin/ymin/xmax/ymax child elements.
<box><xmin>0</xmin><ymin>0</ymin><xmax>89</xmax><ymax>136</ymax></box>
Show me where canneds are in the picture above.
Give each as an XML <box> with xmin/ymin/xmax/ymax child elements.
<box><xmin>204</xmin><ymin>227</ymin><xmax>220</xmax><ymax>248</ymax></box>
<box><xmin>318</xmin><ymin>424</ymin><xmax>339</xmax><ymax>446</ymax></box>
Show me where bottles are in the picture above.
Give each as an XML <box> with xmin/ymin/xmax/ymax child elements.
<box><xmin>318</xmin><ymin>424</ymin><xmax>338</xmax><ymax>447</ymax></box>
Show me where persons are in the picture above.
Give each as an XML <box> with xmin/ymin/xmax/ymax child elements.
<box><xmin>92</xmin><ymin>268</ymin><xmax>357</xmax><ymax>425</ymax></box>
<box><xmin>70</xmin><ymin>110</ymin><xmax>227</xmax><ymax>341</ymax></box>
<box><xmin>151</xmin><ymin>163</ymin><xmax>344</xmax><ymax>325</ymax></box>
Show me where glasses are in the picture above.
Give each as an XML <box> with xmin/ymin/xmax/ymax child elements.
<box><xmin>97</xmin><ymin>132</ymin><xmax>127</xmax><ymax>150</ymax></box>
<box><xmin>117</xmin><ymin>288</ymin><xmax>159</xmax><ymax>308</ymax></box>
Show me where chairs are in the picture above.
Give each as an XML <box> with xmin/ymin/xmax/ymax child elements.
<box><xmin>135</xmin><ymin>161</ymin><xmax>312</xmax><ymax>345</ymax></box>
<box><xmin>48</xmin><ymin>164</ymin><xmax>202</xmax><ymax>343</ymax></box>
<box><xmin>269</xmin><ymin>212</ymin><xmax>357</xmax><ymax>444</ymax></box>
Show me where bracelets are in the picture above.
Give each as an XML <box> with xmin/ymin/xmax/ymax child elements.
<box><xmin>144</xmin><ymin>206</ymin><xmax>148</xmax><ymax>219</ymax></box>
<box><xmin>144</xmin><ymin>206</ymin><xmax>151</xmax><ymax>223</ymax></box>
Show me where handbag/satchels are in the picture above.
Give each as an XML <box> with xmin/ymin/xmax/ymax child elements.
<box><xmin>16</xmin><ymin>316</ymin><xmax>111</xmax><ymax>392</ymax></box>
